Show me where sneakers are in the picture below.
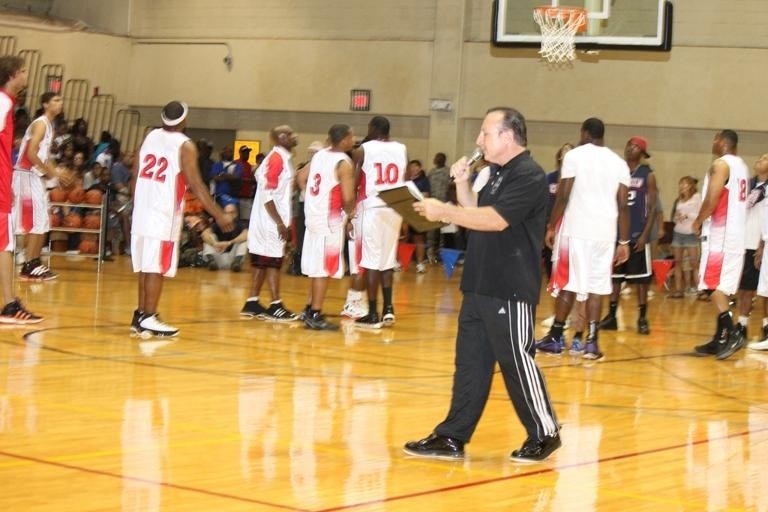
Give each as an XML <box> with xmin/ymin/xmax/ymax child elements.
<box><xmin>694</xmin><ymin>323</ymin><xmax>768</xmax><ymax>360</ymax></box>
<box><xmin>415</xmin><ymin>263</ymin><xmax>425</xmax><ymax>273</ymax></box>
<box><xmin>20</xmin><ymin>258</ymin><xmax>59</xmax><ymax>281</ymax></box>
<box><xmin>0</xmin><ymin>301</ymin><xmax>44</xmax><ymax>324</ymax></box>
<box><xmin>207</xmin><ymin>261</ymin><xmax>220</xmax><ymax>270</ymax></box>
<box><xmin>240</xmin><ymin>302</ymin><xmax>396</xmax><ymax>331</ymax></box>
<box><xmin>130</xmin><ymin>310</ymin><xmax>180</xmax><ymax>339</ymax></box>
<box><xmin>231</xmin><ymin>262</ymin><xmax>241</xmax><ymax>272</ymax></box>
<box><xmin>393</xmin><ymin>263</ymin><xmax>402</xmax><ymax>272</ymax></box>
<box><xmin>638</xmin><ymin>319</ymin><xmax>649</xmax><ymax>335</ymax></box>
<box><xmin>535</xmin><ymin>314</ymin><xmax>617</xmax><ymax>361</ymax></box>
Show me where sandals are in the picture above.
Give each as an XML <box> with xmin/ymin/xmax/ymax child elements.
<box><xmin>668</xmin><ymin>293</ymin><xmax>711</xmax><ymax>301</ymax></box>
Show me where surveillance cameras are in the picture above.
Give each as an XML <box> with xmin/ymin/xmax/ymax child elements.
<box><xmin>223</xmin><ymin>56</ymin><xmax>230</xmax><ymax>65</ymax></box>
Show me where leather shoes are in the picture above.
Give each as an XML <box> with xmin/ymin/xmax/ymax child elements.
<box><xmin>509</xmin><ymin>434</ymin><xmax>561</xmax><ymax>463</ymax></box>
<box><xmin>403</xmin><ymin>434</ymin><xmax>464</xmax><ymax>459</ymax></box>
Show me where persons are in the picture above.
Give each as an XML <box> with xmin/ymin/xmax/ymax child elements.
<box><xmin>533</xmin><ymin>116</ymin><xmax>767</xmax><ymax>361</ymax></box>
<box><xmin>0</xmin><ymin>57</ymin><xmax>453</xmax><ymax>339</ymax></box>
<box><xmin>402</xmin><ymin>108</ymin><xmax>562</xmax><ymax>464</ymax></box>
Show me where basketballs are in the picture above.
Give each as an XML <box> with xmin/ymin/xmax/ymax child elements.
<box><xmin>49</xmin><ymin>214</ymin><xmax>100</xmax><ymax>229</ymax></box>
<box><xmin>49</xmin><ymin>188</ymin><xmax>103</xmax><ymax>205</ymax></box>
<box><xmin>79</xmin><ymin>240</ymin><xmax>97</xmax><ymax>254</ymax></box>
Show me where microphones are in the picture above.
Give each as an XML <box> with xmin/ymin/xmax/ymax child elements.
<box><xmin>447</xmin><ymin>147</ymin><xmax>484</xmax><ymax>184</ymax></box>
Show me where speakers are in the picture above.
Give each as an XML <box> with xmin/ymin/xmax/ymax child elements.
<box><xmin>72</xmin><ymin>18</ymin><xmax>93</xmax><ymax>33</ymax></box>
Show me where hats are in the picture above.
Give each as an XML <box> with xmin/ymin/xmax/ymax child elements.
<box><xmin>629</xmin><ymin>137</ymin><xmax>650</xmax><ymax>158</ymax></box>
<box><xmin>239</xmin><ymin>145</ymin><xmax>252</xmax><ymax>152</ymax></box>
